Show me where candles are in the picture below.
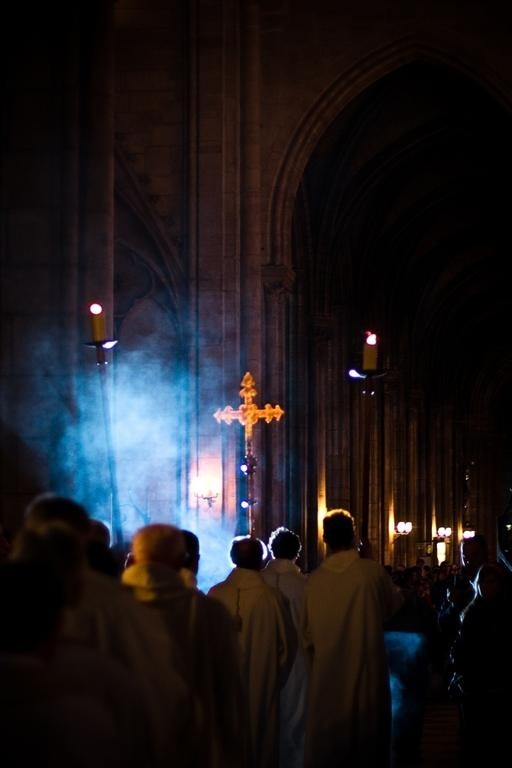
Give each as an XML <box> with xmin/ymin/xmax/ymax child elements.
<box><xmin>90</xmin><ymin>301</ymin><xmax>109</xmax><ymax>342</ymax></box>
<box><xmin>364</xmin><ymin>332</ymin><xmax>379</xmax><ymax>372</ymax></box>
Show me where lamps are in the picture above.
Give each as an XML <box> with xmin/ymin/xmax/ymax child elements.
<box><xmin>393</xmin><ymin>521</ymin><xmax>412</xmax><ymax>543</ymax></box>
<box><xmin>433</xmin><ymin>527</ymin><xmax>454</xmax><ymax>543</ymax></box>
<box><xmin>460</xmin><ymin>530</ymin><xmax>475</xmax><ymax>541</ymax></box>
<box><xmin>240</xmin><ymin>455</ymin><xmax>257</xmax><ymax>479</ymax></box>
<box><xmin>241</xmin><ymin>499</ymin><xmax>256</xmax><ymax>508</ymax></box>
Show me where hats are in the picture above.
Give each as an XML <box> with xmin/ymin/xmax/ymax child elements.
<box><xmin>132</xmin><ymin>525</ymin><xmax>188</xmax><ymax>565</ymax></box>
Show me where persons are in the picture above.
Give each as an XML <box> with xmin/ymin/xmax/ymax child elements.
<box><xmin>395</xmin><ymin>534</ymin><xmax>510</xmax><ymax>768</ymax></box>
<box><xmin>122</xmin><ymin>523</ymin><xmax>240</xmax><ymax>768</ymax></box>
<box><xmin>2</xmin><ymin>496</ymin><xmax>195</xmax><ymax>768</ymax></box>
<box><xmin>300</xmin><ymin>513</ymin><xmax>404</xmax><ymax>768</ymax></box>
<box><xmin>208</xmin><ymin>531</ymin><xmax>309</xmax><ymax>768</ymax></box>
